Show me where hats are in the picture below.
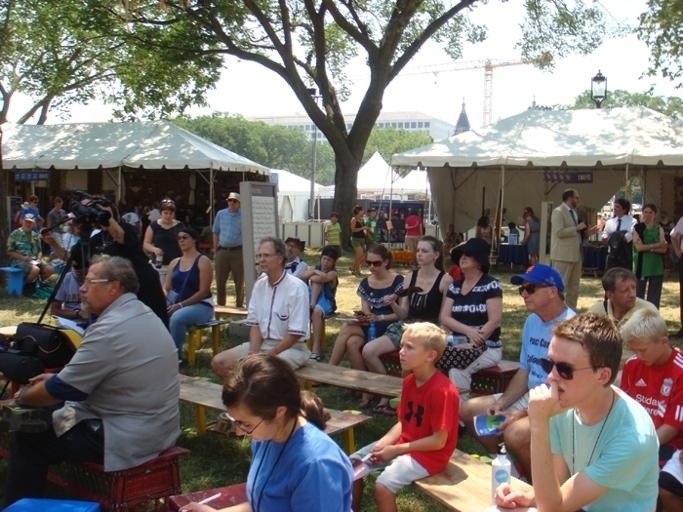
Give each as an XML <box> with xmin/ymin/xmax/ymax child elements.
<box><xmin>449</xmin><ymin>236</ymin><xmax>491</xmax><ymax>272</ymax></box>
<box><xmin>284</xmin><ymin>234</ymin><xmax>307</xmax><ymax>252</ymax></box>
<box><xmin>20</xmin><ymin>201</ymin><xmax>37</xmax><ymax>223</ymax></box>
<box><xmin>510</xmin><ymin>263</ymin><xmax>566</xmax><ymax>290</ymax></box>
<box><xmin>224</xmin><ymin>191</ymin><xmax>243</xmax><ymax>204</ymax></box>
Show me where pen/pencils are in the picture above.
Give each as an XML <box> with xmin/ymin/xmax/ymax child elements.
<box><xmin>181</xmin><ymin>492</ymin><xmax>222</xmax><ymax>512</ymax></box>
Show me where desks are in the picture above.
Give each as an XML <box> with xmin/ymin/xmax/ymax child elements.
<box><xmin>498</xmin><ymin>244</ymin><xmax>529</xmax><ymax>273</ymax></box>
<box><xmin>582</xmin><ymin>244</ymin><xmax>608</xmax><ymax>278</ymax></box>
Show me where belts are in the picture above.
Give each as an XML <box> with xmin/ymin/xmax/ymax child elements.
<box><xmin>218</xmin><ymin>245</ymin><xmax>243</xmax><ymax>251</ymax></box>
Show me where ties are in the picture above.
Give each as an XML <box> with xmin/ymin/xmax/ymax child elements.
<box><xmin>616</xmin><ymin>219</ymin><xmax>622</xmax><ymax>231</ymax></box>
<box><xmin>569</xmin><ymin>209</ymin><xmax>579</xmax><ymax>234</ymax></box>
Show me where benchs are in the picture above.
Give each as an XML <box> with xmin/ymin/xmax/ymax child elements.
<box><xmin>2</xmin><ymin>497</ymin><xmax>100</xmax><ymax>512</ymax></box>
<box><xmin>165</xmin><ymin>477</ymin><xmax>247</xmax><ymax>512</ymax></box>
<box><xmin>0</xmin><ymin>266</ymin><xmax>27</xmax><ymax>297</ymax></box>
<box><xmin>187</xmin><ymin>319</ymin><xmax>234</xmax><ymax>370</ymax></box>
<box><xmin>412</xmin><ymin>448</ymin><xmax>540</xmax><ymax>512</ymax></box>
<box><xmin>175</xmin><ymin>373</ymin><xmax>371</xmax><ymax>456</ymax></box>
<box><xmin>379</xmin><ymin>351</ymin><xmax>521</xmax><ymax>396</ymax></box>
<box><xmin>293</xmin><ymin>358</ymin><xmax>402</xmax><ymax>399</ymax></box>
<box><xmin>214</xmin><ymin>306</ymin><xmax>341</xmax><ymax>349</ymax></box>
<box><xmin>45</xmin><ymin>440</ymin><xmax>190</xmax><ymax>512</ymax></box>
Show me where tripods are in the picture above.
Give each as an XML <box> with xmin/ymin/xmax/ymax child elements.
<box><xmin>0</xmin><ymin>216</ymin><xmax>98</xmax><ymax>402</ymax></box>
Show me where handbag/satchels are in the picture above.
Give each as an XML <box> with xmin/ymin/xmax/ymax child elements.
<box><xmin>164</xmin><ymin>287</ymin><xmax>182</xmax><ymax>308</ymax></box>
<box><xmin>1</xmin><ymin>316</ymin><xmax>82</xmax><ymax>385</ymax></box>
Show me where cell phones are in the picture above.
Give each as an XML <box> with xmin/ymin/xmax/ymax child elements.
<box><xmin>354</xmin><ymin>310</ymin><xmax>366</xmax><ymax>316</ymax></box>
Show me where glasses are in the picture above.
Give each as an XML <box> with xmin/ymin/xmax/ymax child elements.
<box><xmin>175</xmin><ymin>234</ymin><xmax>193</xmax><ymax>242</ymax></box>
<box><xmin>364</xmin><ymin>254</ymin><xmax>387</xmax><ymax>268</ymax></box>
<box><xmin>518</xmin><ymin>282</ymin><xmax>554</xmax><ymax>296</ymax></box>
<box><xmin>229</xmin><ymin>198</ymin><xmax>239</xmax><ymax>204</ymax></box>
<box><xmin>254</xmin><ymin>252</ymin><xmax>279</xmax><ymax>260</ymax></box>
<box><xmin>158</xmin><ymin>199</ymin><xmax>176</xmax><ymax>208</ymax></box>
<box><xmin>221</xmin><ymin>403</ymin><xmax>273</xmax><ymax>438</ymax></box>
<box><xmin>537</xmin><ymin>352</ymin><xmax>607</xmax><ymax>379</ymax></box>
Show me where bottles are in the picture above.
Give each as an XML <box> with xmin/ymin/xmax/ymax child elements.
<box><xmin>156</xmin><ymin>253</ymin><xmax>164</xmax><ymax>269</ymax></box>
<box><xmin>490</xmin><ymin>442</ymin><xmax>512</xmax><ymax>505</ymax></box>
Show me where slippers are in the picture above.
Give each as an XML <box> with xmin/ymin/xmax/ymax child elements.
<box><xmin>308</xmin><ymin>349</ymin><xmax>326</xmax><ymax>365</ymax></box>
<box><xmin>357</xmin><ymin>389</ymin><xmax>397</xmax><ymax>415</ymax></box>
